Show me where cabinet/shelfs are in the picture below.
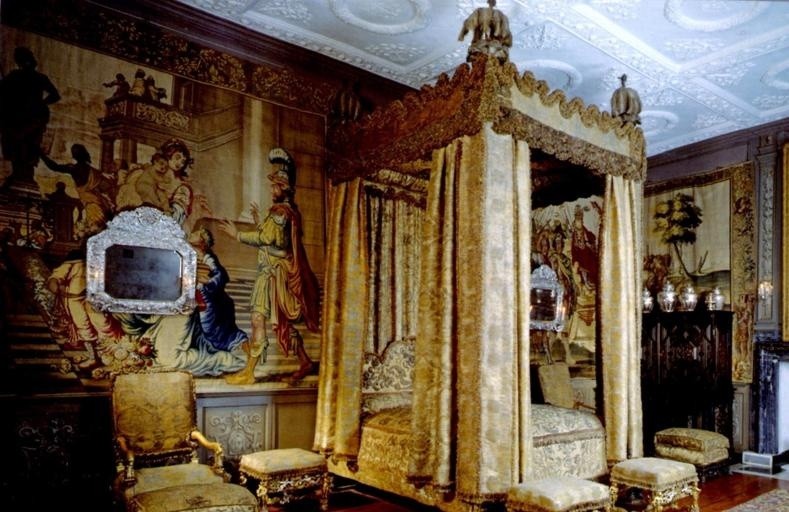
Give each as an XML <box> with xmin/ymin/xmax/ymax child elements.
<box><xmin>641</xmin><ymin>310</ymin><xmax>733</xmax><ymax>458</ymax></box>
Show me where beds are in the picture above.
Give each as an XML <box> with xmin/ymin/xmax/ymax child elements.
<box><xmin>316</xmin><ymin>0</ymin><xmax>648</xmax><ymax>512</ymax></box>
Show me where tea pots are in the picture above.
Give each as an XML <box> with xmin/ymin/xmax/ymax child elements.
<box><xmin>642</xmin><ymin>277</ymin><xmax>726</xmax><ymax>313</ymax></box>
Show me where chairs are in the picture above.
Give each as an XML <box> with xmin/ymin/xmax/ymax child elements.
<box><xmin>109</xmin><ymin>367</ymin><xmax>231</xmax><ymax>497</ymax></box>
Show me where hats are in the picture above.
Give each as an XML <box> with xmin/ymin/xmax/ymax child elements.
<box><xmin>268</xmin><ymin>171</ymin><xmax>290</xmax><ymax>190</ymax></box>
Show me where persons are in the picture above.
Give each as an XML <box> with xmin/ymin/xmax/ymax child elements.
<box><xmin>532</xmin><ymin>212</ymin><xmax>598</xmax><ymax>284</ymax></box>
<box><xmin>0</xmin><ymin>45</ymin><xmax>322</xmax><ymax>384</ymax></box>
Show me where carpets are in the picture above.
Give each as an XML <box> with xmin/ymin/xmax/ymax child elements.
<box><xmin>724</xmin><ymin>487</ymin><xmax>788</xmax><ymax>512</ymax></box>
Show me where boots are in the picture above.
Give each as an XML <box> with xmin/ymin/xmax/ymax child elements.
<box><xmin>226</xmin><ymin>355</ymin><xmax>258</xmax><ymax>384</ymax></box>
<box><xmin>293</xmin><ymin>346</ymin><xmax>313</xmax><ymax>378</ymax></box>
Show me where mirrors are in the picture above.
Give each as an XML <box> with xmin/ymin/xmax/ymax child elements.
<box><xmin>530</xmin><ymin>264</ymin><xmax>564</xmax><ymax>332</ymax></box>
<box><xmin>85</xmin><ymin>206</ymin><xmax>198</xmax><ymax>315</ymax></box>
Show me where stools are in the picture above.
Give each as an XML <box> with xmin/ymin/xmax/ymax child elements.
<box><xmin>505</xmin><ymin>478</ymin><xmax>611</xmax><ymax>512</ymax></box>
<box><xmin>239</xmin><ymin>447</ymin><xmax>332</xmax><ymax>512</ymax></box>
<box><xmin>127</xmin><ymin>483</ymin><xmax>258</xmax><ymax>512</ymax></box>
<box><xmin>609</xmin><ymin>457</ymin><xmax>701</xmax><ymax>512</ymax></box>
